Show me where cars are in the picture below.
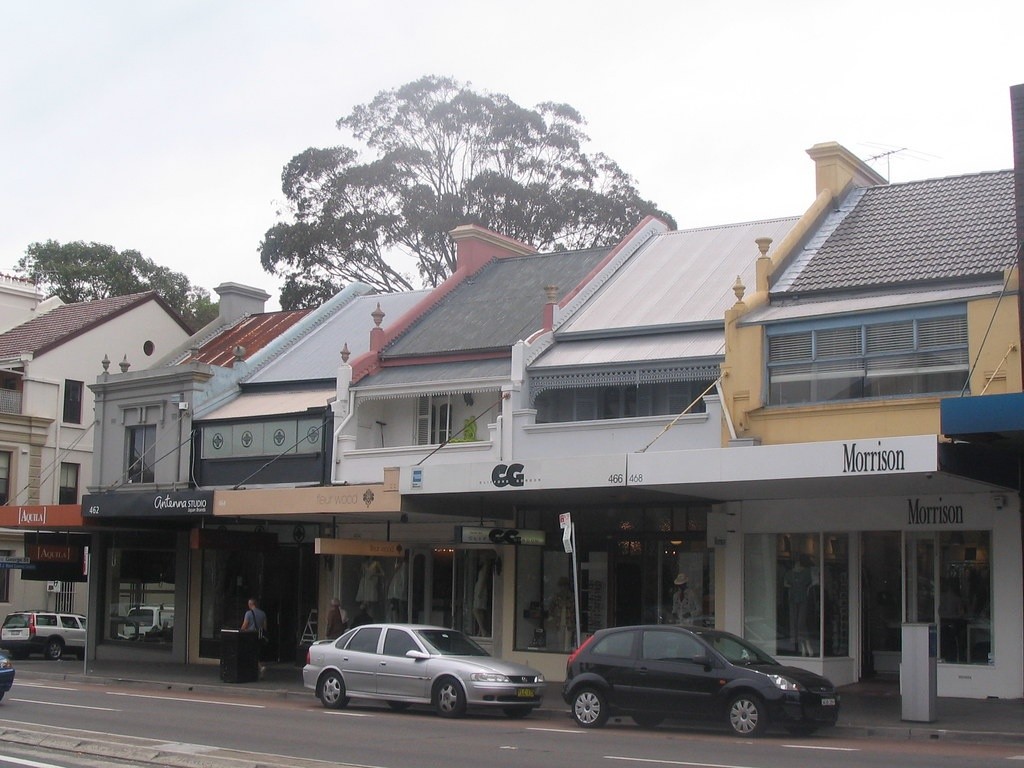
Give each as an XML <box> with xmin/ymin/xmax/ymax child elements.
<box><xmin>302</xmin><ymin>623</ymin><xmax>547</xmax><ymax>719</ymax></box>
<box><xmin>0</xmin><ymin>654</ymin><xmax>16</xmax><ymax>701</ymax></box>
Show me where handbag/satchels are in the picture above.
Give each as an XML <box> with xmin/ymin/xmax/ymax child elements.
<box><xmin>258</xmin><ymin>627</ymin><xmax>273</xmax><ymax>647</ymax></box>
<box><xmin>339</xmin><ymin>607</ymin><xmax>349</xmax><ymax>624</ymax></box>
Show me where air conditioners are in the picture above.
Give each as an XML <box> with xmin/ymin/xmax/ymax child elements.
<box><xmin>46</xmin><ymin>581</ymin><xmax>62</xmax><ymax>593</ymax></box>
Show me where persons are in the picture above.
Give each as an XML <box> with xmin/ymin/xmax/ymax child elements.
<box><xmin>326</xmin><ymin>598</ymin><xmax>373</xmax><ymax>640</ymax></box>
<box><xmin>241</xmin><ymin>599</ymin><xmax>267</xmax><ymax>680</ymax></box>
<box><xmin>355</xmin><ymin>557</ymin><xmax>406</xmax><ymax>623</ymax></box>
<box><xmin>555</xmin><ymin>576</ymin><xmax>575</xmax><ymax>654</ymax></box>
<box><xmin>783</xmin><ymin>561</ymin><xmax>814</xmax><ymax>657</ymax></box>
<box><xmin>671</xmin><ymin>573</ymin><xmax>699</xmax><ymax>624</ymax></box>
<box><xmin>472</xmin><ymin>568</ymin><xmax>488</xmax><ymax>636</ymax></box>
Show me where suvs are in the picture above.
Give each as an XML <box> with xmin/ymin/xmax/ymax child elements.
<box><xmin>123</xmin><ymin>604</ymin><xmax>175</xmax><ymax>641</ymax></box>
<box><xmin>559</xmin><ymin>622</ymin><xmax>842</xmax><ymax>737</ymax></box>
<box><xmin>0</xmin><ymin>609</ymin><xmax>87</xmax><ymax>661</ymax></box>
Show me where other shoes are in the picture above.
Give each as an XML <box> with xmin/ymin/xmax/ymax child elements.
<box><xmin>260</xmin><ymin>666</ymin><xmax>266</xmax><ymax>680</ymax></box>
